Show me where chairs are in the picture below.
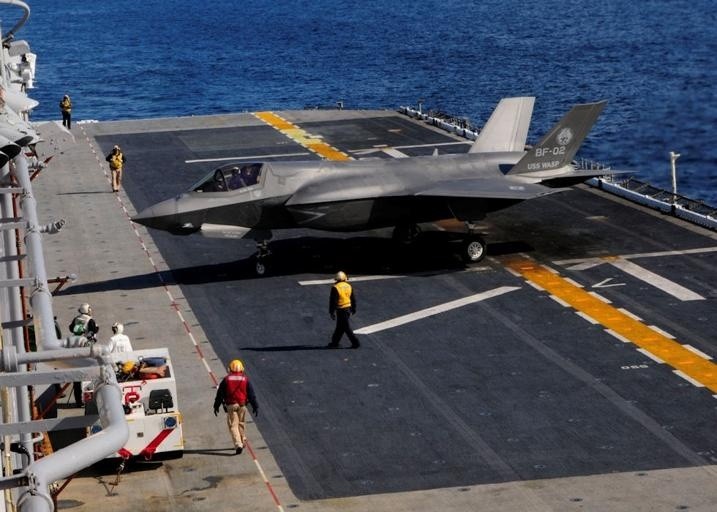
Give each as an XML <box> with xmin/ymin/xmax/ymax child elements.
<box><xmin>150</xmin><ymin>389</ymin><xmax>174</xmax><ymax>413</ymax></box>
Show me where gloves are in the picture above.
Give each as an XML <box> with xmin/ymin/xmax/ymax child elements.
<box><xmin>252</xmin><ymin>407</ymin><xmax>259</xmax><ymax>417</ymax></box>
<box><xmin>214</xmin><ymin>408</ymin><xmax>219</xmax><ymax>417</ymax></box>
<box><xmin>352</xmin><ymin>307</ymin><xmax>357</xmax><ymax>315</ymax></box>
<box><xmin>331</xmin><ymin>313</ymin><xmax>336</xmax><ymax>321</ymax></box>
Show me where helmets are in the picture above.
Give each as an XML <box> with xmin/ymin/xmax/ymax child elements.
<box><xmin>228</xmin><ymin>359</ymin><xmax>245</xmax><ymax>373</ymax></box>
<box><xmin>112</xmin><ymin>323</ymin><xmax>124</xmax><ymax>334</ymax></box>
<box><xmin>73</xmin><ymin>324</ymin><xmax>85</xmax><ymax>335</ymax></box>
<box><xmin>79</xmin><ymin>302</ymin><xmax>91</xmax><ymax>314</ymax></box>
<box><xmin>122</xmin><ymin>360</ymin><xmax>134</xmax><ymax>374</ymax></box>
<box><xmin>336</xmin><ymin>271</ymin><xmax>347</xmax><ymax>282</ymax></box>
<box><xmin>112</xmin><ymin>145</ymin><xmax>120</xmax><ymax>151</ymax></box>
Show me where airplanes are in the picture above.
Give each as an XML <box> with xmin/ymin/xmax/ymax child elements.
<box><xmin>123</xmin><ymin>88</ymin><xmax>654</xmax><ymax>279</ymax></box>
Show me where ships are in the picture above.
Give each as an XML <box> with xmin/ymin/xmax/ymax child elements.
<box><xmin>1</xmin><ymin>1</ymin><xmax>716</xmax><ymax>512</ymax></box>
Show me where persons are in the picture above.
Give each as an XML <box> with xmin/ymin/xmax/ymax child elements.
<box><xmin>62</xmin><ymin>324</ymin><xmax>91</xmax><ymax>408</ymax></box>
<box><xmin>327</xmin><ymin>270</ymin><xmax>359</xmax><ymax>349</ymax></box>
<box><xmin>59</xmin><ymin>95</ymin><xmax>71</xmax><ymax>129</ymax></box>
<box><xmin>215</xmin><ymin>360</ymin><xmax>259</xmax><ymax>454</ymax></box>
<box><xmin>106</xmin><ymin>145</ymin><xmax>127</xmax><ymax>192</ymax></box>
<box><xmin>229</xmin><ymin>168</ymin><xmax>246</xmax><ymax>189</ymax></box>
<box><xmin>69</xmin><ymin>304</ymin><xmax>99</xmax><ymax>344</ymax></box>
<box><xmin>108</xmin><ymin>322</ymin><xmax>133</xmax><ymax>352</ymax></box>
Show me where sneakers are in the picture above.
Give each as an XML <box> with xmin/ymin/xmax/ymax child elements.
<box><xmin>351</xmin><ymin>342</ymin><xmax>360</xmax><ymax>349</ymax></box>
<box><xmin>235</xmin><ymin>445</ymin><xmax>243</xmax><ymax>455</ymax></box>
<box><xmin>328</xmin><ymin>341</ymin><xmax>339</xmax><ymax>347</ymax></box>
